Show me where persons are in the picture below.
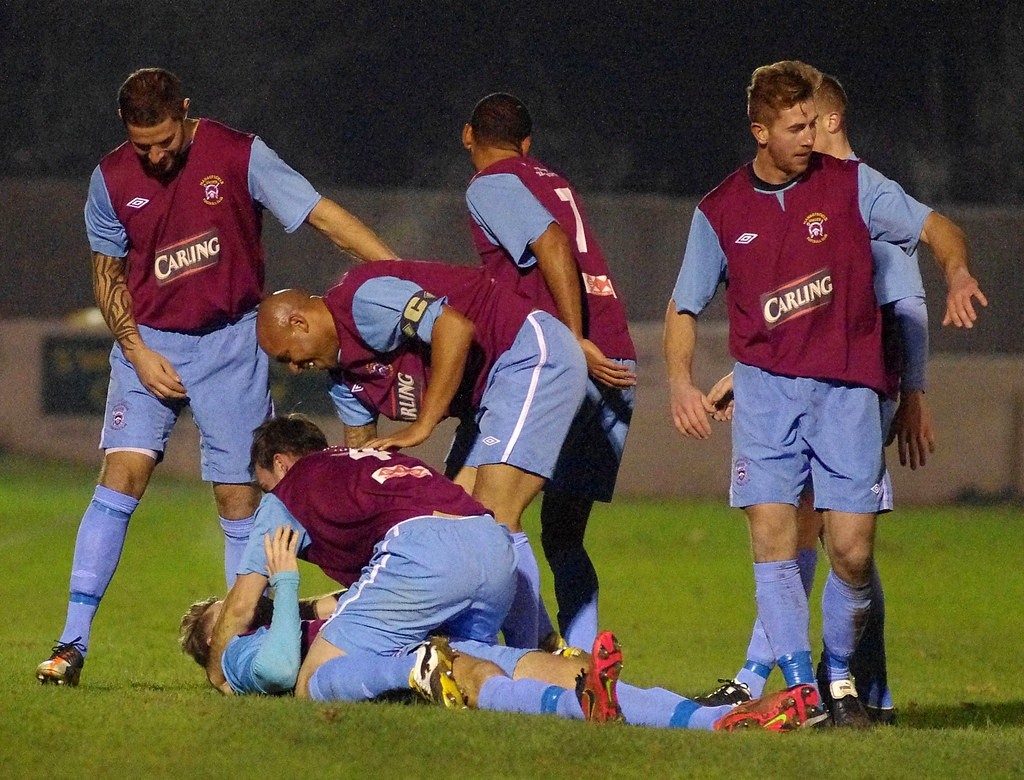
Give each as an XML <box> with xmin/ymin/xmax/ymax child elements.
<box><xmin>662</xmin><ymin>60</ymin><xmax>988</xmax><ymax>730</ymax></box>
<box><xmin>256</xmin><ymin>260</ymin><xmax>589</xmax><ymax>657</ymax></box>
<box><xmin>34</xmin><ymin>67</ymin><xmax>404</xmax><ymax>688</ymax></box>
<box><xmin>179</xmin><ymin>411</ymin><xmax>830</xmax><ymax>731</ymax></box>
<box><xmin>463</xmin><ymin>92</ymin><xmax>639</xmax><ymax>654</ymax></box>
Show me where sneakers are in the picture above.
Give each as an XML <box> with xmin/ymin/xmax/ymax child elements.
<box><xmin>817</xmin><ymin>665</ymin><xmax>870</xmax><ymax>729</ymax></box>
<box><xmin>34</xmin><ymin>637</ymin><xmax>88</xmax><ymax>687</ymax></box>
<box><xmin>574</xmin><ymin>631</ymin><xmax>630</xmax><ymax>726</ymax></box>
<box><xmin>408</xmin><ymin>639</ymin><xmax>465</xmax><ymax>709</ymax></box>
<box><xmin>552</xmin><ymin>647</ymin><xmax>590</xmax><ymax>659</ymax></box>
<box><xmin>693</xmin><ymin>677</ymin><xmax>753</xmax><ymax>707</ymax></box>
<box><xmin>855</xmin><ymin>670</ymin><xmax>898</xmax><ymax>727</ymax></box>
<box><xmin>713</xmin><ymin>684</ymin><xmax>826</xmax><ymax>734</ymax></box>
